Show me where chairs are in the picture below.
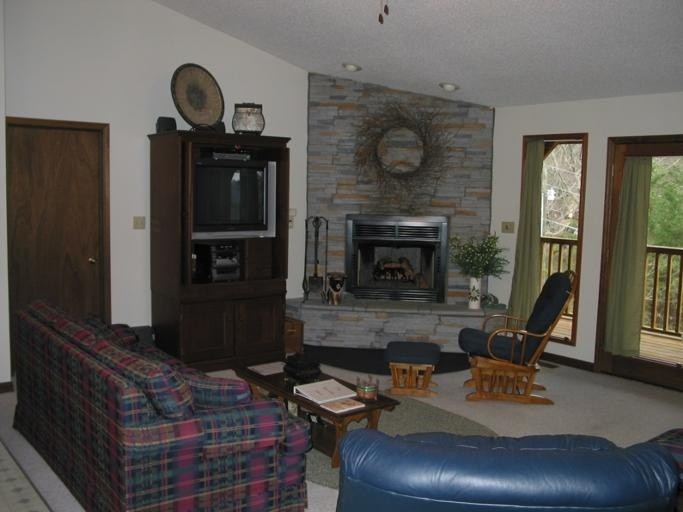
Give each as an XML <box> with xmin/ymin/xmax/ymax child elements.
<box><xmin>337</xmin><ymin>425</ymin><xmax>680</xmax><ymax>512</ymax></box>
<box><xmin>454</xmin><ymin>270</ymin><xmax>577</xmax><ymax>405</ymax></box>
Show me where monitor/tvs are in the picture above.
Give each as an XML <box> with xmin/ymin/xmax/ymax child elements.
<box><xmin>191</xmin><ymin>160</ymin><xmax>268</xmax><ymax>233</ymax></box>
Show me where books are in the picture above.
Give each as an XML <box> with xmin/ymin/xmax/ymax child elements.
<box><xmin>319</xmin><ymin>397</ymin><xmax>365</xmax><ymax>414</ymax></box>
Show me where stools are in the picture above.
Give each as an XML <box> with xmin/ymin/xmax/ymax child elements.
<box><xmin>383</xmin><ymin>338</ymin><xmax>441</xmax><ymax>399</ymax></box>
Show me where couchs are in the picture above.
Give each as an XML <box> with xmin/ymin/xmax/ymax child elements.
<box><xmin>11</xmin><ymin>297</ymin><xmax>311</xmax><ymax>511</ymax></box>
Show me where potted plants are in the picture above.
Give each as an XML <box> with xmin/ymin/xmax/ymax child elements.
<box><xmin>448</xmin><ymin>232</ymin><xmax>509</xmax><ymax>311</ymax></box>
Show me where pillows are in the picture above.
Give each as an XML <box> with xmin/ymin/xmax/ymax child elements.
<box><xmin>178</xmin><ymin>366</ymin><xmax>253</xmax><ymax>414</ymax></box>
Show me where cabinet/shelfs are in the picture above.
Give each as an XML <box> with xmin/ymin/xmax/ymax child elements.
<box><xmin>147</xmin><ymin>130</ymin><xmax>288</xmax><ymax>373</ymax></box>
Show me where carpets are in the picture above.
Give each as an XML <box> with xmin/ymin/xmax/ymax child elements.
<box><xmin>286</xmin><ymin>390</ymin><xmax>498</xmax><ymax>491</ymax></box>
